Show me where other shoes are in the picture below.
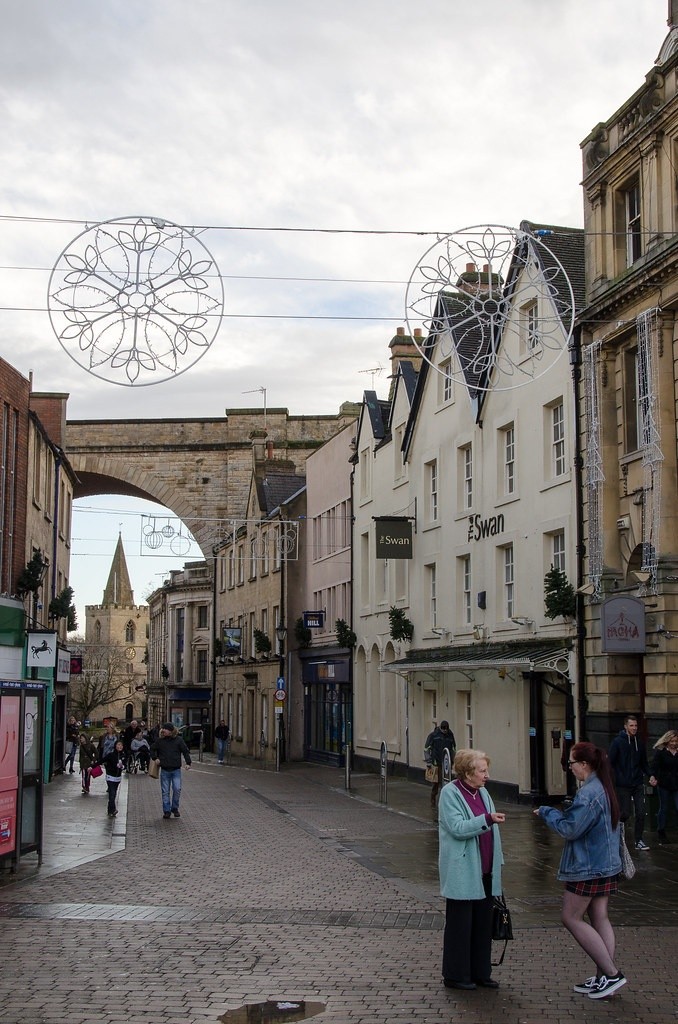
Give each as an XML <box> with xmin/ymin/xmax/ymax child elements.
<box><xmin>431</xmin><ymin>803</ymin><xmax>436</xmax><ymax>809</ymax></box>
<box><xmin>69</xmin><ymin>769</ymin><xmax>75</xmax><ymax>772</ymax></box>
<box><xmin>171</xmin><ymin>807</ymin><xmax>180</xmax><ymax>817</ymax></box>
<box><xmin>108</xmin><ymin>813</ymin><xmax>114</xmax><ymax>817</ymax></box>
<box><xmin>163</xmin><ymin>812</ymin><xmax>171</xmax><ymax>818</ymax></box>
<box><xmin>113</xmin><ymin>811</ymin><xmax>118</xmax><ymax>815</ymax></box>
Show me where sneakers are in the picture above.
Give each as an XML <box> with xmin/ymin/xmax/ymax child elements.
<box><xmin>634</xmin><ymin>840</ymin><xmax>650</xmax><ymax>850</ymax></box>
<box><xmin>588</xmin><ymin>971</ymin><xmax>627</xmax><ymax>999</ymax></box>
<box><xmin>574</xmin><ymin>975</ymin><xmax>615</xmax><ymax>995</ymax></box>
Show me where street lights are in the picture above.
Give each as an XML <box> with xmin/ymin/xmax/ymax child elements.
<box><xmin>275</xmin><ymin>620</ymin><xmax>286</xmax><ymax>764</ymax></box>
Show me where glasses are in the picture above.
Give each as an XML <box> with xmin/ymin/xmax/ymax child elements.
<box><xmin>568</xmin><ymin>760</ymin><xmax>588</xmax><ymax>765</ymax></box>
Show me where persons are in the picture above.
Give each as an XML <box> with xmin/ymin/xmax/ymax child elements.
<box><xmin>215</xmin><ymin>720</ymin><xmax>230</xmax><ymax>764</ymax></box>
<box><xmin>96</xmin><ymin>722</ymin><xmax>120</xmax><ymax>791</ymax></box>
<box><xmin>424</xmin><ymin>720</ymin><xmax>457</xmax><ymax>807</ymax></box>
<box><xmin>65</xmin><ymin>716</ymin><xmax>81</xmax><ymax>773</ymax></box>
<box><xmin>89</xmin><ymin>739</ymin><xmax>127</xmax><ymax>816</ymax></box>
<box><xmin>533</xmin><ymin>740</ymin><xmax>627</xmax><ymax>999</ymax></box>
<box><xmin>122</xmin><ymin>720</ymin><xmax>140</xmax><ymax>769</ymax></box>
<box><xmin>79</xmin><ymin>733</ymin><xmax>99</xmax><ymax>794</ymax></box>
<box><xmin>151</xmin><ymin>724</ymin><xmax>160</xmax><ymax>737</ymax></box>
<box><xmin>150</xmin><ymin>722</ymin><xmax>192</xmax><ymax>819</ymax></box>
<box><xmin>606</xmin><ymin>715</ymin><xmax>657</xmax><ymax>851</ymax></box>
<box><xmin>651</xmin><ymin>730</ymin><xmax>678</xmax><ymax>845</ymax></box>
<box><xmin>140</xmin><ymin>721</ymin><xmax>149</xmax><ymax>735</ymax></box>
<box><xmin>131</xmin><ymin>733</ymin><xmax>150</xmax><ymax>771</ymax></box>
<box><xmin>438</xmin><ymin>750</ymin><xmax>506</xmax><ymax>990</ymax></box>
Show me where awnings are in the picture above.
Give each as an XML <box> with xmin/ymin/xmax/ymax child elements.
<box><xmin>169</xmin><ymin>687</ymin><xmax>212</xmax><ymax>702</ymax></box>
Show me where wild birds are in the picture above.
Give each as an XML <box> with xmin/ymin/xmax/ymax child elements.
<box><xmin>225</xmin><ymin>636</ymin><xmax>240</xmax><ymax>648</ymax></box>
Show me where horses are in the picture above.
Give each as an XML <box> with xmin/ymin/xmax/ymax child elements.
<box><xmin>31</xmin><ymin>640</ymin><xmax>53</xmax><ymax>658</ymax></box>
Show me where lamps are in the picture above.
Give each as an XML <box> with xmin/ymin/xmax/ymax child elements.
<box><xmin>472</xmin><ymin>623</ymin><xmax>487</xmax><ymax>639</ymax></box>
<box><xmin>430</xmin><ymin>627</ymin><xmax>451</xmax><ymax>635</ymax></box>
<box><xmin>510</xmin><ymin>617</ymin><xmax>534</xmax><ymax>625</ymax></box>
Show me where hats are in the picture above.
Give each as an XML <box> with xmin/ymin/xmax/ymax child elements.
<box><xmin>162</xmin><ymin>722</ymin><xmax>173</xmax><ymax>731</ymax></box>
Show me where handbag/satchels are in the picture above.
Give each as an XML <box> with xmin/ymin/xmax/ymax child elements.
<box><xmin>425</xmin><ymin>766</ymin><xmax>439</xmax><ymax>783</ymax></box>
<box><xmin>148</xmin><ymin>743</ymin><xmax>159</xmax><ymax>779</ymax></box>
<box><xmin>619</xmin><ymin>822</ymin><xmax>636</xmax><ymax>880</ymax></box>
<box><xmin>65</xmin><ymin>741</ymin><xmax>73</xmax><ymax>753</ymax></box>
<box><xmin>90</xmin><ymin>766</ymin><xmax>103</xmax><ymax>778</ymax></box>
<box><xmin>492</xmin><ymin>892</ymin><xmax>514</xmax><ymax>940</ymax></box>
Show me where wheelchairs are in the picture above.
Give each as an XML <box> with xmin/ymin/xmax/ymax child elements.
<box><xmin>125</xmin><ymin>750</ymin><xmax>150</xmax><ymax>774</ymax></box>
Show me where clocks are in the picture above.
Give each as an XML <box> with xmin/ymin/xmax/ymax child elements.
<box><xmin>125</xmin><ymin>647</ymin><xmax>136</xmax><ymax>660</ymax></box>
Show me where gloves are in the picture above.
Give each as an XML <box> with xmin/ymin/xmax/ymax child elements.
<box><xmin>427</xmin><ymin>762</ymin><xmax>432</xmax><ymax>768</ymax></box>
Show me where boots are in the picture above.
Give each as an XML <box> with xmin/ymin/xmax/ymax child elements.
<box><xmin>656</xmin><ymin>829</ymin><xmax>671</xmax><ymax>845</ymax></box>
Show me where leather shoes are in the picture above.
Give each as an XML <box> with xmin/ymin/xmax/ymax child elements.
<box><xmin>472</xmin><ymin>977</ymin><xmax>499</xmax><ymax>987</ymax></box>
<box><xmin>444</xmin><ymin>978</ymin><xmax>475</xmax><ymax>989</ymax></box>
